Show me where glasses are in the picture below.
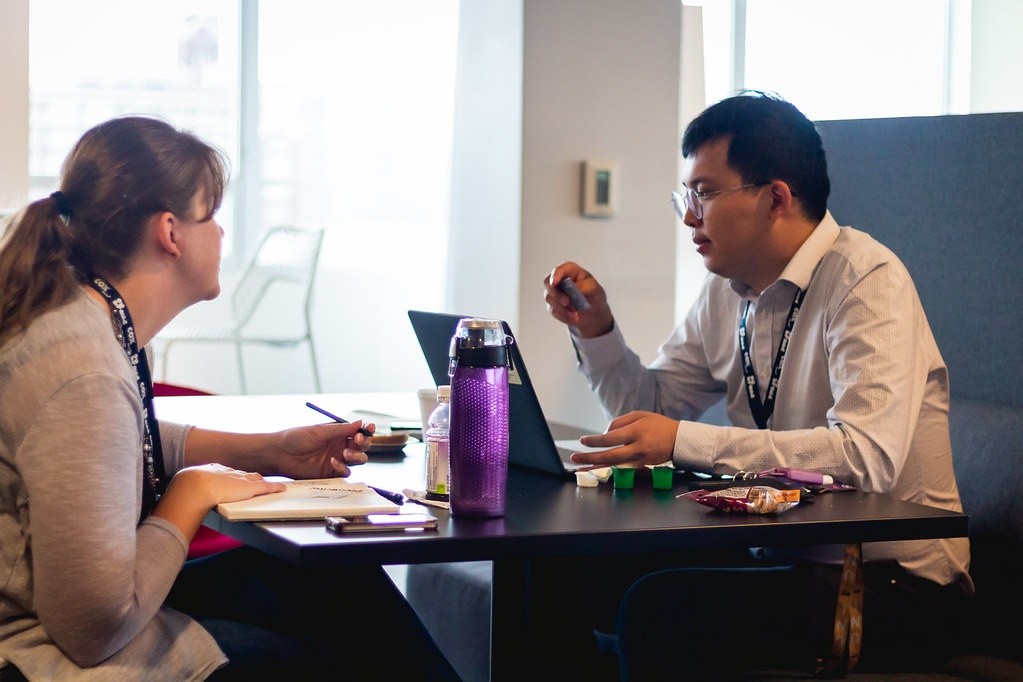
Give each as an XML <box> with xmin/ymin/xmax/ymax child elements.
<box><xmin>671</xmin><ymin>182</ymin><xmax>796</xmax><ymax>220</ymax></box>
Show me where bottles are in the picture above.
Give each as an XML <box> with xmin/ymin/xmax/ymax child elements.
<box><xmin>425</xmin><ymin>385</ymin><xmax>452</xmax><ymax>503</ymax></box>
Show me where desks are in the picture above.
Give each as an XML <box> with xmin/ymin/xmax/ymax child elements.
<box><xmin>153</xmin><ymin>392</ymin><xmax>973</xmax><ymax>682</ymax></box>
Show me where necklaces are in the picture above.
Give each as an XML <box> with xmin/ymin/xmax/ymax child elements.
<box><xmin>110</xmin><ymin>304</ymin><xmax>161</xmax><ymax>502</ymax></box>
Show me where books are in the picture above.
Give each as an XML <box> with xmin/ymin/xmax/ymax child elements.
<box><xmin>210</xmin><ymin>475</ymin><xmax>429</xmax><ymax>546</ymax></box>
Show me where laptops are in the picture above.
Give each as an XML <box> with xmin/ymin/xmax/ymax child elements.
<box><xmin>409</xmin><ymin>310</ymin><xmax>625</xmax><ymax>477</ymax></box>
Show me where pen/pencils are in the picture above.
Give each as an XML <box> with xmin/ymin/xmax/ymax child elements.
<box><xmin>307</xmin><ymin>402</ymin><xmax>374</xmax><ymax>438</ymax></box>
<box><xmin>369</xmin><ymin>487</ymin><xmax>404</xmax><ymax>504</ymax></box>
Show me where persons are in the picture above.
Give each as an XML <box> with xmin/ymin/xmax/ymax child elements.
<box><xmin>0</xmin><ymin>116</ymin><xmax>376</xmax><ymax>682</ymax></box>
<box><xmin>544</xmin><ymin>96</ymin><xmax>971</xmax><ymax>682</ymax></box>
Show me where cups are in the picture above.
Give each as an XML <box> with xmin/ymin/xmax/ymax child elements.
<box><xmin>447</xmin><ymin>318</ymin><xmax>514</xmax><ymax>520</ymax></box>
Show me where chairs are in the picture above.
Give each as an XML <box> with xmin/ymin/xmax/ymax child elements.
<box><xmin>155</xmin><ymin>223</ymin><xmax>326</xmax><ymax>392</ymax></box>
<box><xmin>739</xmin><ymin>398</ymin><xmax>1023</xmax><ymax>682</ymax></box>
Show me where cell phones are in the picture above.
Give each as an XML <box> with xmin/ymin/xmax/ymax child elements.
<box><xmin>324</xmin><ymin>512</ymin><xmax>438</xmax><ymax>534</ymax></box>
